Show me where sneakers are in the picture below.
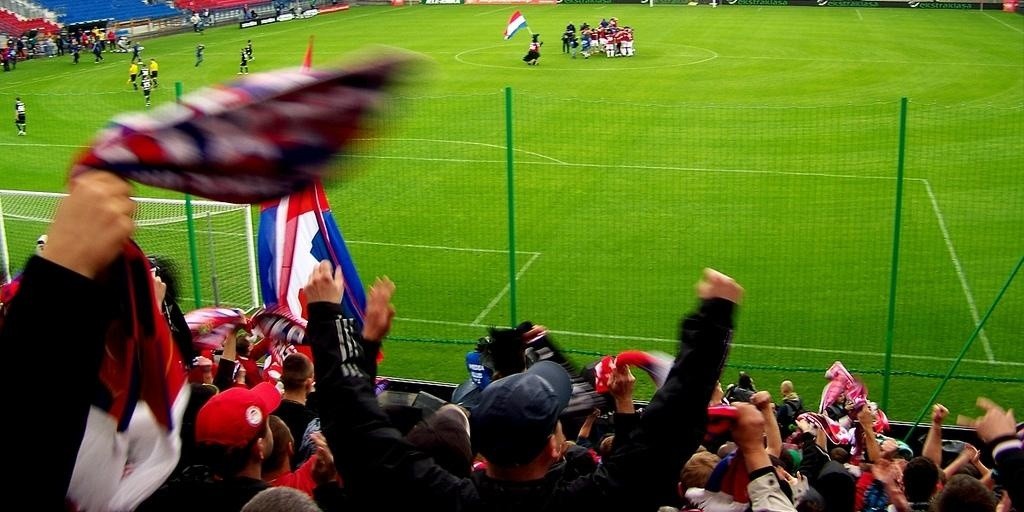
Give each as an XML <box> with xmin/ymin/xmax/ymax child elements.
<box><xmin>17</xmin><ymin>130</ymin><xmax>26</xmax><ymax>136</ymax></box>
<box><xmin>49</xmin><ymin>49</ymin><xmax>253</xmax><ymax>108</ymax></box>
<box><xmin>528</xmin><ymin>50</ymin><xmax>635</xmax><ymax>63</ymax></box>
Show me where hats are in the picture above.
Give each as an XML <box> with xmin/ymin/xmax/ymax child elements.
<box><xmin>194</xmin><ymin>380</ymin><xmax>283</xmax><ymax>450</ymax></box>
<box><xmin>470</xmin><ymin>357</ymin><xmax>575</xmax><ymax>466</ymax></box>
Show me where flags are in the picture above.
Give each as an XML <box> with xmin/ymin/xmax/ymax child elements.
<box><xmin>504</xmin><ymin>11</ymin><xmax>527</xmax><ymax>40</ymax></box>
<box><xmin>257</xmin><ymin>38</ymin><xmax>368</xmax><ymax>329</ymax></box>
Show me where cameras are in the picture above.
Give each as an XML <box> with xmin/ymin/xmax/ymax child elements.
<box><xmin>726</xmin><ymin>369</ymin><xmax>755</xmax><ymax>403</ymax></box>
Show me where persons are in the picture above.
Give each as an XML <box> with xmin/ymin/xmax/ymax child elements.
<box><xmin>0</xmin><ymin>22</ymin><xmax>145</xmax><ymax>71</ymax></box>
<box><xmin>522</xmin><ymin>16</ymin><xmax>635</xmax><ymax>66</ymax></box>
<box><xmin>138</xmin><ymin>63</ymin><xmax>149</xmax><ymax>77</ymax></box>
<box><xmin>141</xmin><ymin>75</ymin><xmax>153</xmax><ymax>106</ymax></box>
<box><xmin>246</xmin><ymin>39</ymin><xmax>254</xmax><ymax>62</ymax></box>
<box><xmin>128</xmin><ymin>61</ymin><xmax>139</xmax><ymax>91</ymax></box>
<box><xmin>14</xmin><ymin>96</ymin><xmax>26</xmax><ymax>136</ymax></box>
<box><xmin>189</xmin><ymin>0</ymin><xmax>339</xmax><ymax>33</ymax></box>
<box><xmin>149</xmin><ymin>58</ymin><xmax>159</xmax><ymax>88</ymax></box>
<box><xmin>194</xmin><ymin>42</ymin><xmax>204</xmax><ymax>69</ymax></box>
<box><xmin>238</xmin><ymin>49</ymin><xmax>249</xmax><ymax>76</ymax></box>
